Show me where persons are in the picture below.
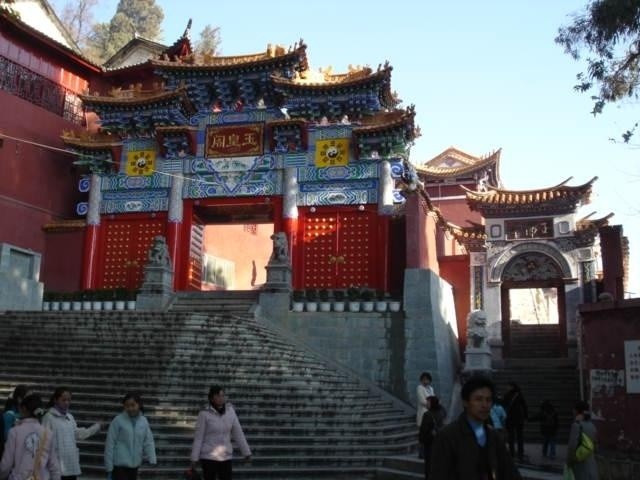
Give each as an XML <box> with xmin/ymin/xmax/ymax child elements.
<box><xmin>102</xmin><ymin>392</ymin><xmax>158</xmax><ymax>479</ymax></box>
<box><xmin>0</xmin><ymin>384</ymin><xmax>35</xmax><ymax>450</ymax></box>
<box><xmin>564</xmin><ymin>399</ymin><xmax>600</xmax><ymax>480</ymax></box>
<box><xmin>430</xmin><ymin>375</ymin><xmax>523</xmax><ymax>479</ymax></box>
<box><xmin>0</xmin><ymin>393</ymin><xmax>62</xmax><ymax>480</ymax></box>
<box><xmin>42</xmin><ymin>386</ymin><xmax>106</xmax><ymax>479</ymax></box>
<box><xmin>527</xmin><ymin>399</ymin><xmax>562</xmax><ymax>460</ymax></box>
<box><xmin>414</xmin><ymin>371</ymin><xmax>437</xmax><ymax>460</ymax></box>
<box><xmin>500</xmin><ymin>379</ymin><xmax>530</xmax><ymax>463</ymax></box>
<box><xmin>488</xmin><ymin>392</ymin><xmax>508</xmax><ymax>447</ymax></box>
<box><xmin>189</xmin><ymin>383</ymin><xmax>253</xmax><ymax>480</ymax></box>
<box><xmin>419</xmin><ymin>395</ymin><xmax>447</xmax><ymax>479</ymax></box>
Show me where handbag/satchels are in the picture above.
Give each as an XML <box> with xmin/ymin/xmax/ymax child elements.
<box><xmin>574</xmin><ymin>424</ymin><xmax>594</xmax><ymax>464</ymax></box>
